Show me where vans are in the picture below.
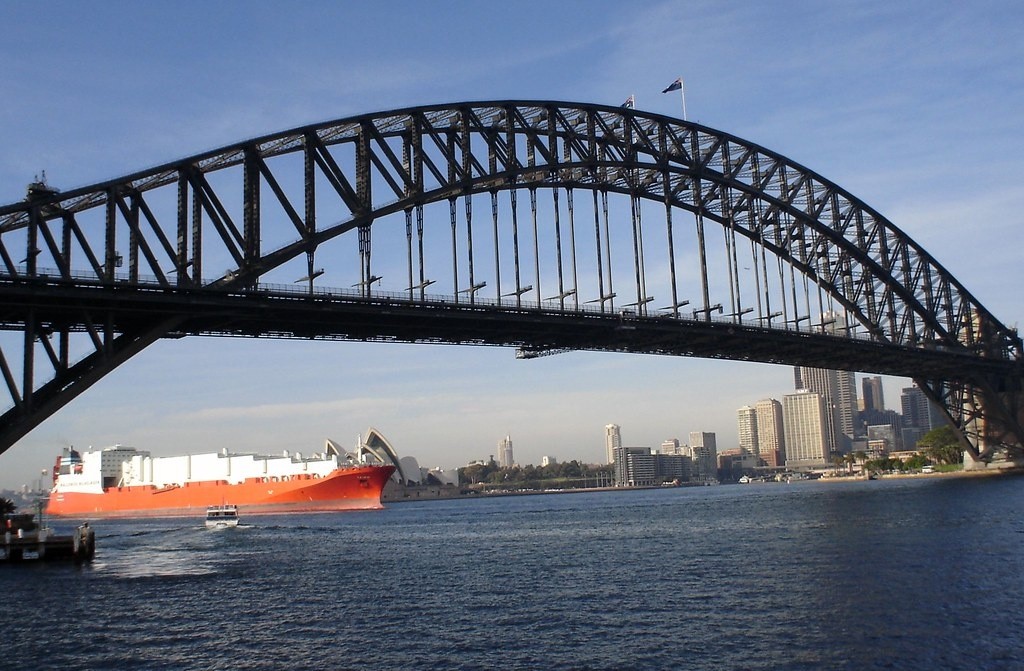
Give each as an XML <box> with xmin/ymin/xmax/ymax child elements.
<box><xmin>922</xmin><ymin>466</ymin><xmax>934</xmax><ymax>474</ymax></box>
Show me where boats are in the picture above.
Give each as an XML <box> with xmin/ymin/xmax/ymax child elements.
<box><xmin>205</xmin><ymin>501</ymin><xmax>239</xmax><ymax>531</ymax></box>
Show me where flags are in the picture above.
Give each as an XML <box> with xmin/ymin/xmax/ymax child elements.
<box><xmin>619</xmin><ymin>96</ymin><xmax>634</xmax><ymax>110</ymax></box>
<box><xmin>661</xmin><ymin>76</ymin><xmax>682</xmax><ymax>94</ymax></box>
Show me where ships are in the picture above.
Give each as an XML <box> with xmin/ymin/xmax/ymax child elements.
<box><xmin>45</xmin><ymin>445</ymin><xmax>397</xmax><ymax>519</ymax></box>
